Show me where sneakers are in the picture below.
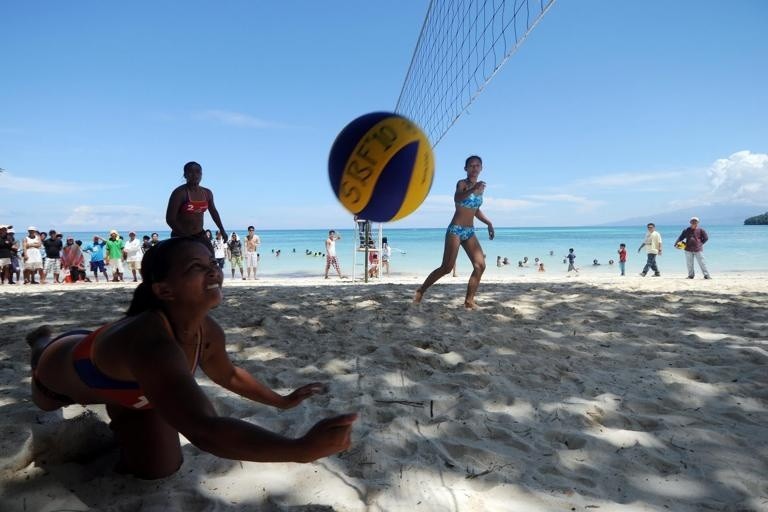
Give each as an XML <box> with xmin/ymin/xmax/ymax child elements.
<box><xmin>686</xmin><ymin>275</ymin><xmax>693</xmax><ymax>279</ymax></box>
<box><xmin>640</xmin><ymin>272</ymin><xmax>646</xmax><ymax>278</ymax></box>
<box><xmin>704</xmin><ymin>275</ymin><xmax>712</xmax><ymax>279</ymax></box>
<box><xmin>651</xmin><ymin>274</ymin><xmax>661</xmax><ymax>276</ymax></box>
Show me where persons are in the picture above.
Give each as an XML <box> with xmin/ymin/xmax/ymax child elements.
<box><xmin>0</xmin><ymin>224</ymin><xmax>260</xmax><ymax>285</ymax></box>
<box><xmin>27</xmin><ymin>239</ymin><xmax>357</xmax><ymax>480</ymax></box>
<box><xmin>617</xmin><ymin>244</ymin><xmax>628</xmax><ymax>276</ymax></box>
<box><xmin>676</xmin><ymin>215</ymin><xmax>711</xmax><ymax>280</ymax></box>
<box><xmin>452</xmin><ymin>264</ymin><xmax>457</xmax><ymax>277</ymax></box>
<box><xmin>258</xmin><ymin>247</ymin><xmax>326</xmax><ymax>260</ymax></box>
<box><xmin>498</xmin><ymin>247</ymin><xmax>615</xmax><ymax>271</ymax></box>
<box><xmin>169</xmin><ymin>161</ymin><xmax>228</xmax><ymax>254</ymax></box>
<box><xmin>352</xmin><ymin>215</ymin><xmax>391</xmax><ymax>278</ymax></box>
<box><xmin>412</xmin><ymin>155</ymin><xmax>494</xmax><ymax>311</ymax></box>
<box><xmin>638</xmin><ymin>223</ymin><xmax>664</xmax><ymax>278</ymax></box>
<box><xmin>323</xmin><ymin>231</ymin><xmax>348</xmax><ymax>281</ymax></box>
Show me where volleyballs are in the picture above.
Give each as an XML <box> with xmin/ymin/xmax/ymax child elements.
<box><xmin>676</xmin><ymin>241</ymin><xmax>686</xmax><ymax>250</ymax></box>
<box><xmin>329</xmin><ymin>112</ymin><xmax>435</xmax><ymax>222</ymax></box>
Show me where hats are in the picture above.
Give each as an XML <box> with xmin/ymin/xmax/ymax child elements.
<box><xmin>0</xmin><ymin>225</ymin><xmax>15</xmax><ymax>235</ymax></box>
<box><xmin>67</xmin><ymin>236</ymin><xmax>73</xmax><ymax>240</ymax></box>
<box><xmin>27</xmin><ymin>227</ymin><xmax>37</xmax><ymax>232</ymax></box>
<box><xmin>690</xmin><ymin>217</ymin><xmax>699</xmax><ymax>222</ymax></box>
<box><xmin>56</xmin><ymin>232</ymin><xmax>62</xmax><ymax>237</ymax></box>
